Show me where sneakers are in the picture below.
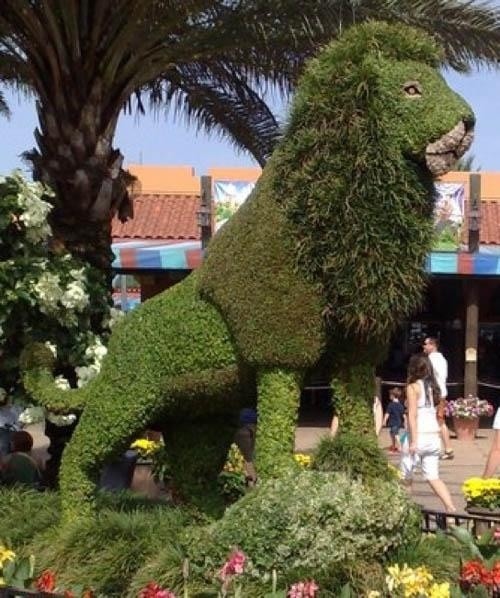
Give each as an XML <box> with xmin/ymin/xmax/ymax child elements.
<box><xmin>442</xmin><ymin>450</ymin><xmax>454</xmax><ymax>459</ymax></box>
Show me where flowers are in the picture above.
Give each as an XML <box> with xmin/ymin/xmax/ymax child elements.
<box><xmin>461</xmin><ymin>476</ymin><xmax>500</xmax><ymax>508</ymax></box>
<box><xmin>444</xmin><ymin>392</ymin><xmax>494</xmax><ymax>420</ymax></box>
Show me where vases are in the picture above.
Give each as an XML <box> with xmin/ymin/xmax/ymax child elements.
<box><xmin>468</xmin><ymin>507</ymin><xmax>499</xmax><ymax>537</ymax></box>
<box><xmin>452</xmin><ymin>417</ymin><xmax>480</xmax><ymax>442</ymax></box>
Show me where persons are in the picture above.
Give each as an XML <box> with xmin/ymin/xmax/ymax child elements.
<box><xmin>0</xmin><ymin>431</ymin><xmax>42</xmax><ymax>488</ymax></box>
<box><xmin>421</xmin><ymin>336</ymin><xmax>453</xmax><ymax>462</ymax></box>
<box><xmin>383</xmin><ymin>386</ymin><xmax>407</xmax><ymax>453</ymax></box>
<box><xmin>397</xmin><ymin>349</ymin><xmax>457</xmax><ymax>512</ymax></box>
<box><xmin>483</xmin><ymin>406</ymin><xmax>500</xmax><ymax>480</ymax></box>
